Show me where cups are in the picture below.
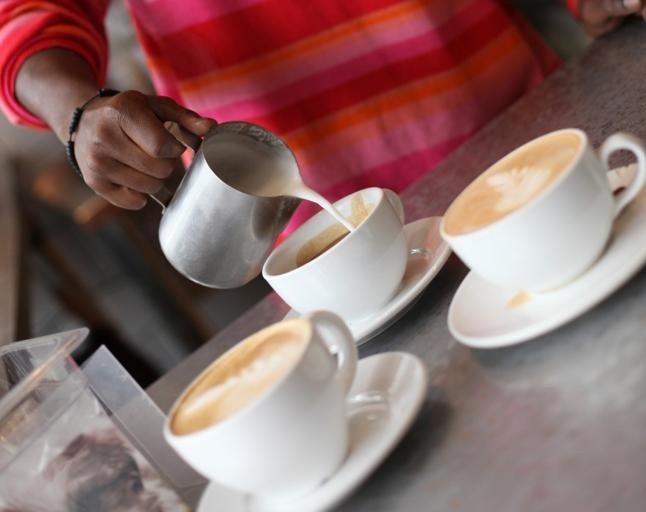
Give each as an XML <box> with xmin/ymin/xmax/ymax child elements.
<box><xmin>437</xmin><ymin>124</ymin><xmax>645</xmax><ymax>295</ymax></box>
<box><xmin>147</xmin><ymin>117</ymin><xmax>302</xmax><ymax>291</ymax></box>
<box><xmin>261</xmin><ymin>187</ymin><xmax>411</xmax><ymax>326</ymax></box>
<box><xmin>162</xmin><ymin>307</ymin><xmax>360</xmax><ymax>499</ymax></box>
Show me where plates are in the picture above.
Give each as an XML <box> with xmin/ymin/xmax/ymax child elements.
<box><xmin>282</xmin><ymin>214</ymin><xmax>453</xmax><ymax>342</ymax></box>
<box><xmin>196</xmin><ymin>350</ymin><xmax>427</xmax><ymax>510</ymax></box>
<box><xmin>446</xmin><ymin>162</ymin><xmax>644</xmax><ymax>349</ymax></box>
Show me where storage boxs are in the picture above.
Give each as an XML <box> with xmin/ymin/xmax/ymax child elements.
<box><xmin>0</xmin><ymin>328</ymin><xmax>191</xmax><ymax>512</ymax></box>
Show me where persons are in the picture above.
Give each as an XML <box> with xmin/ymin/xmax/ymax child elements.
<box><xmin>0</xmin><ymin>0</ymin><xmax>645</xmax><ymax>210</ymax></box>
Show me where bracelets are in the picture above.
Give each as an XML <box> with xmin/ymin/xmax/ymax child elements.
<box><xmin>66</xmin><ymin>88</ymin><xmax>119</xmax><ymax>179</ymax></box>
